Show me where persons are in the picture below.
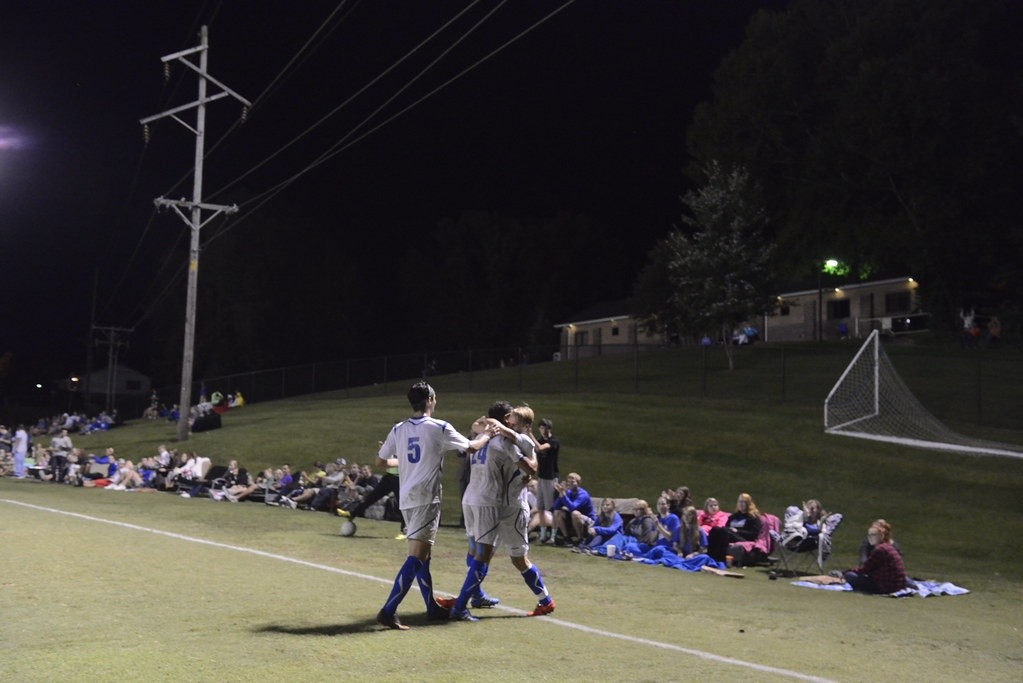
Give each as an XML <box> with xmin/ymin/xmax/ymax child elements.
<box><xmin>376</xmin><ymin>380</ymin><xmax>500</xmax><ymax>630</ymax></box>
<box><xmin>731</xmin><ymin>322</ymin><xmax>759</xmax><ymax>345</ymax></box>
<box><xmin>700</xmin><ymin>331</ymin><xmax>712</xmax><ymax>348</ymax></box>
<box><xmin>839</xmin><ymin>320</ymin><xmax>849</xmax><ymax>340</ymax></box>
<box><xmin>959</xmin><ymin>305</ymin><xmax>1003</xmax><ymax>346</ymax></box>
<box><xmin>451</xmin><ymin>399</ymin><xmax>538</xmax><ymax>624</ymax></box>
<box><xmin>707</xmin><ymin>493</ymin><xmax>763</xmax><ymax>566</ymax></box>
<box><xmin>435</xmin><ymin>404</ymin><xmax>555</xmax><ymax>617</ymax></box>
<box><xmin>47</xmin><ymin>427</ymin><xmax>73</xmax><ymax>483</ymax></box>
<box><xmin>0</xmin><ymin>388</ymin><xmax>906</xmax><ymax>596</ymax></box>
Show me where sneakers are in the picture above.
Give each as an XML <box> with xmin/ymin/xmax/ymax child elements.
<box><xmin>436</xmin><ymin>595</ymin><xmax>457</xmax><ymax>608</ymax></box>
<box><xmin>425</xmin><ymin>607</ymin><xmax>450</xmax><ymax>621</ymax></box>
<box><xmin>527</xmin><ymin>600</ymin><xmax>555</xmax><ymax>616</ymax></box>
<box><xmin>471</xmin><ymin>594</ymin><xmax>499</xmax><ymax>608</ymax></box>
<box><xmin>377</xmin><ymin>609</ymin><xmax>410</xmax><ymax>630</ymax></box>
<box><xmin>449</xmin><ymin>605</ymin><xmax>480</xmax><ymax>621</ymax></box>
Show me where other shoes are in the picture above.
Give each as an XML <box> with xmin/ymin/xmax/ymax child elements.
<box><xmin>114</xmin><ymin>484</ymin><xmax>127</xmax><ymax>490</ymax></box>
<box><xmin>104</xmin><ymin>483</ymin><xmax>118</xmax><ymax>490</ymax></box>
<box><xmin>207</xmin><ymin>486</ymin><xmax>238</xmax><ymax>503</ymax></box>
<box><xmin>551</xmin><ymin>538</ymin><xmax>575</xmax><ymax>547</ymax></box>
<box><xmin>395</xmin><ymin>531</ymin><xmax>408</xmax><ymax>541</ymax></box>
<box><xmin>336</xmin><ymin>508</ymin><xmax>355</xmax><ymax>522</ymax></box>
<box><xmin>265</xmin><ymin>495</ymin><xmax>297</xmax><ymax>509</ymax></box>
<box><xmin>536</xmin><ymin>537</ymin><xmax>554</xmax><ymax>546</ymax></box>
<box><xmin>568</xmin><ymin>539</ymin><xmax>584</xmax><ymax>547</ymax></box>
<box><xmin>783</xmin><ymin>533</ymin><xmax>804</xmax><ymax>547</ymax></box>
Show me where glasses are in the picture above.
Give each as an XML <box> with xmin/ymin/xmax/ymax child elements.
<box><xmin>866</xmin><ymin>533</ymin><xmax>880</xmax><ymax>538</ymax></box>
